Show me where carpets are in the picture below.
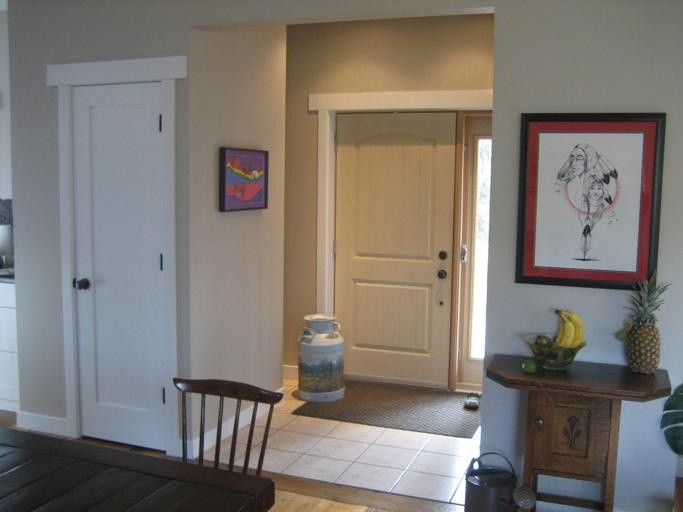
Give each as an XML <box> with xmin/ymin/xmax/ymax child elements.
<box><xmin>291</xmin><ymin>380</ymin><xmax>481</xmax><ymax>438</ymax></box>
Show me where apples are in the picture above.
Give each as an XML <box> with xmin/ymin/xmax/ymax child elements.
<box><xmin>534</xmin><ymin>335</ymin><xmax>573</xmax><ymax>367</ymax></box>
<box><xmin>521</xmin><ymin>359</ymin><xmax>538</xmax><ymax>374</ymax></box>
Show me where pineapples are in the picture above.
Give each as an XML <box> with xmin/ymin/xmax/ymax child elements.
<box><xmin>625</xmin><ymin>273</ymin><xmax>671</xmax><ymax>373</ymax></box>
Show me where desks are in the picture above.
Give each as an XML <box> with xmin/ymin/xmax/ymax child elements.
<box><xmin>1</xmin><ymin>422</ymin><xmax>275</xmax><ymax>512</ymax></box>
<box><xmin>487</xmin><ymin>351</ymin><xmax>672</xmax><ymax>511</ymax></box>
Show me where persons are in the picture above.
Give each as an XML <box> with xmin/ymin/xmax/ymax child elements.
<box><xmin>553</xmin><ymin>142</ymin><xmax>618</xmax><ymax>259</ymax></box>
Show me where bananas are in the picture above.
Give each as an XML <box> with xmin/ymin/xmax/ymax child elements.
<box><xmin>552</xmin><ymin>308</ymin><xmax>583</xmax><ymax>349</ymax></box>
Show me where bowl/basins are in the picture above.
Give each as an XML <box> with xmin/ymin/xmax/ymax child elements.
<box><xmin>522</xmin><ymin>331</ymin><xmax>584</xmax><ymax>369</ymax></box>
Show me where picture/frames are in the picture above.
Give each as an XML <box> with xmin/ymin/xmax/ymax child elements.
<box><xmin>516</xmin><ymin>112</ymin><xmax>666</xmax><ymax>295</ymax></box>
<box><xmin>220</xmin><ymin>146</ymin><xmax>269</xmax><ymax>213</ymax></box>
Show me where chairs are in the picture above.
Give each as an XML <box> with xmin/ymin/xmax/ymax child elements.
<box><xmin>173</xmin><ymin>379</ymin><xmax>283</xmax><ymax>474</ymax></box>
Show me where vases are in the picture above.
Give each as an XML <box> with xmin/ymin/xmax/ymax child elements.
<box><xmin>297</xmin><ymin>314</ymin><xmax>345</xmax><ymax>402</ymax></box>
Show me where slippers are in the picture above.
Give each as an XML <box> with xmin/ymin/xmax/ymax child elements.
<box><xmin>464</xmin><ymin>390</ymin><xmax>480</xmax><ymax>409</ymax></box>
